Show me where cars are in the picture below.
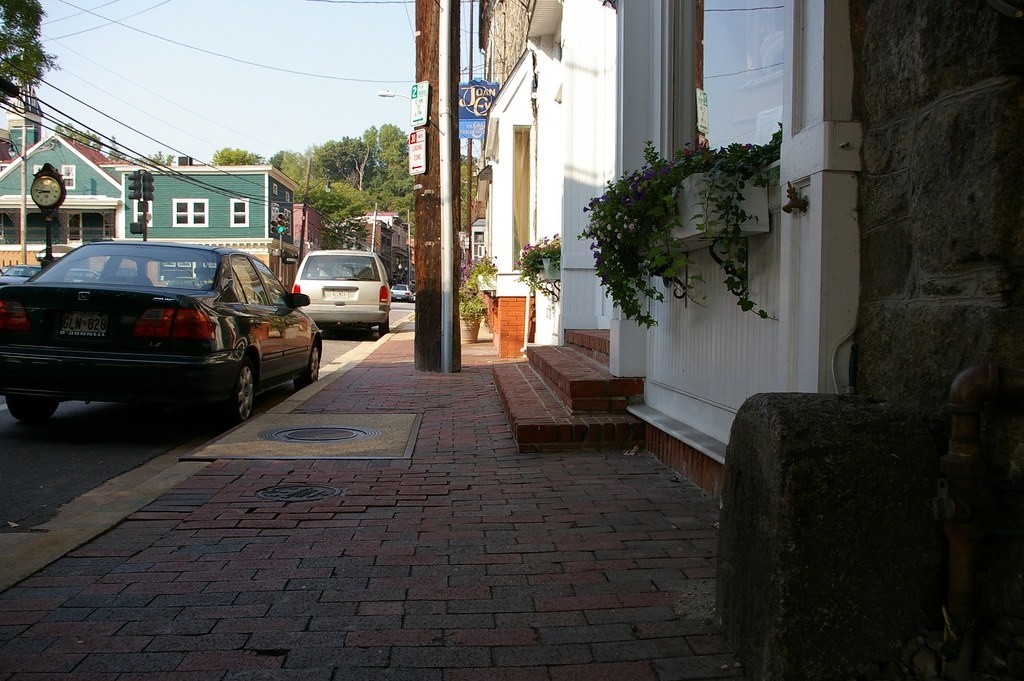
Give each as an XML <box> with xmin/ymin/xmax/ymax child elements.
<box><xmin>0</xmin><ymin>240</ymin><xmax>324</xmax><ymax>425</ymax></box>
<box><xmin>290</xmin><ymin>249</ymin><xmax>397</xmax><ymax>336</ymax></box>
<box><xmin>66</xmin><ymin>268</ymin><xmax>101</xmax><ymax>283</ymax></box>
<box><xmin>390</xmin><ymin>284</ymin><xmax>416</xmax><ymax>303</ymax></box>
<box><xmin>0</xmin><ymin>264</ymin><xmax>41</xmax><ymax>285</ymax></box>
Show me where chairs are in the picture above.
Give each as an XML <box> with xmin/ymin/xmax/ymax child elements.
<box><xmin>353</xmin><ymin>267</ymin><xmax>373</xmax><ymax>279</ymax></box>
<box><xmin>319</xmin><ymin>267</ymin><xmax>334</xmax><ymax>280</ymax></box>
<box><xmin>111</xmin><ymin>268</ymin><xmax>153</xmax><ymax>287</ymax></box>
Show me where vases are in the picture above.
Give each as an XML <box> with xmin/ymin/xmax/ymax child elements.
<box><xmin>550</xmin><ymin>256</ymin><xmax>560</xmax><ymax>272</ymax></box>
<box><xmin>459</xmin><ymin>314</ymin><xmax>482</xmax><ymax>342</ymax></box>
<box><xmin>644</xmin><ymin>171</ymin><xmax>769</xmax><ymax>258</ymax></box>
<box><xmin>525</xmin><ymin>261</ymin><xmax>543</xmax><ymax>275</ymax></box>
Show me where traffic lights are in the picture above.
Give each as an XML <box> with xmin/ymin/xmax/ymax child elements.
<box><xmin>130</xmin><ymin>222</ymin><xmax>144</xmax><ymax>236</ymax></box>
<box><xmin>143</xmin><ymin>172</ymin><xmax>155</xmax><ymax>201</ymax></box>
<box><xmin>128</xmin><ymin>170</ymin><xmax>141</xmax><ymax>200</ymax></box>
<box><xmin>278</xmin><ymin>213</ymin><xmax>286</xmax><ymax>234</ymax></box>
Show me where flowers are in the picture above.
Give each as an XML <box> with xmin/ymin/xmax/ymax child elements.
<box><xmin>576</xmin><ymin>135</ymin><xmax>778</xmax><ymax>325</ymax></box>
<box><xmin>542</xmin><ymin>234</ymin><xmax>560</xmax><ymax>276</ymax></box>
<box><xmin>515</xmin><ymin>241</ymin><xmax>543</xmax><ymax>290</ymax></box>
<box><xmin>459</xmin><ymin>281</ymin><xmax>487</xmax><ymax>328</ymax></box>
<box><xmin>462</xmin><ymin>256</ymin><xmax>498</xmax><ymax>280</ymax></box>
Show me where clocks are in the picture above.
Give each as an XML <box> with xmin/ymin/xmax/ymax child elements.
<box><xmin>30</xmin><ymin>176</ymin><xmax>61</xmax><ymax>207</ymax></box>
